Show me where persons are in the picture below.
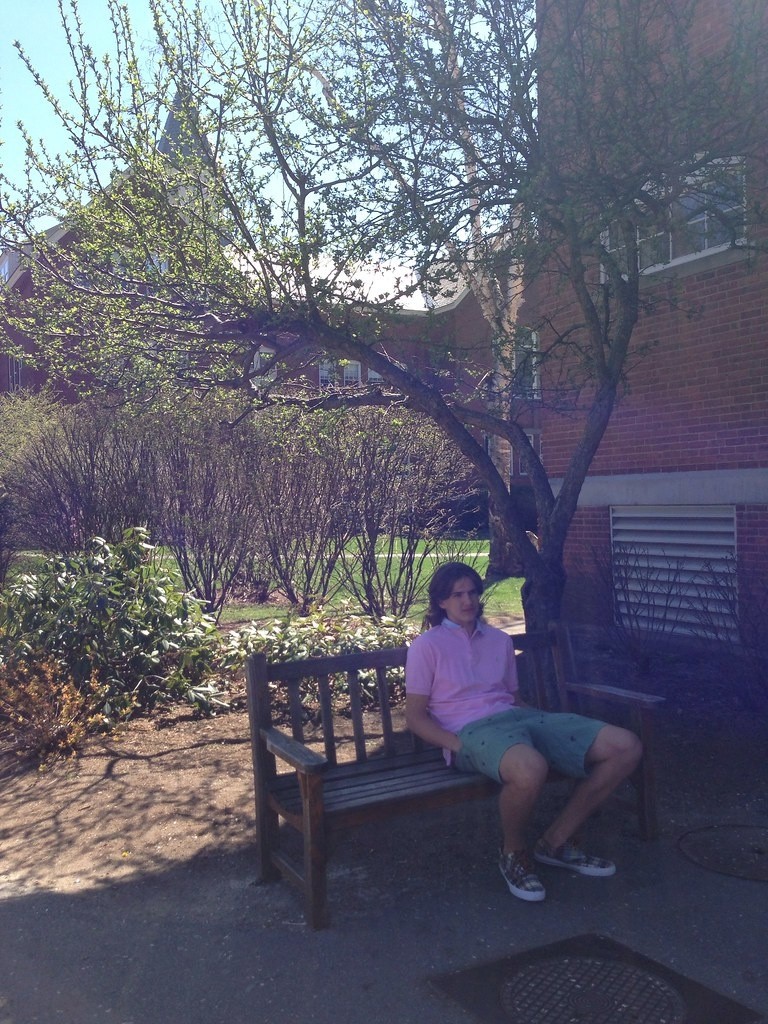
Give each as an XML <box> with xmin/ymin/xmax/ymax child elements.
<box><xmin>406</xmin><ymin>560</ymin><xmax>644</xmax><ymax>901</ymax></box>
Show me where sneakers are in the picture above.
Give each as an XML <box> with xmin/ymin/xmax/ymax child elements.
<box><xmin>498</xmin><ymin>846</ymin><xmax>545</xmax><ymax>901</ymax></box>
<box><xmin>533</xmin><ymin>837</ymin><xmax>616</xmax><ymax>877</ymax></box>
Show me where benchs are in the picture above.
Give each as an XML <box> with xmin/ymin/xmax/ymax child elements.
<box><xmin>246</xmin><ymin>618</ymin><xmax>666</xmax><ymax>928</ymax></box>
